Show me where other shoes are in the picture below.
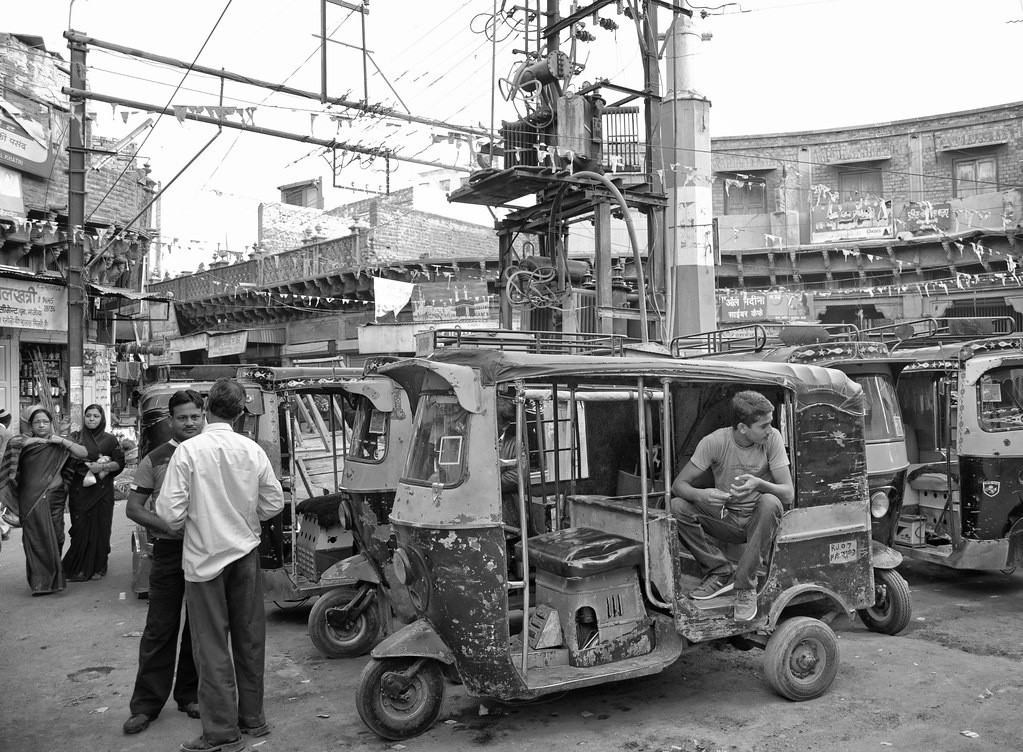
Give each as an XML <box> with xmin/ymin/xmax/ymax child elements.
<box><xmin>178</xmin><ymin>701</ymin><xmax>200</xmax><ymax>717</ymax></box>
<box><xmin>180</xmin><ymin>730</ymin><xmax>244</xmax><ymax>752</ymax></box>
<box><xmin>239</xmin><ymin>722</ymin><xmax>270</xmax><ymax>738</ymax></box>
<box><xmin>124</xmin><ymin>713</ymin><xmax>150</xmax><ymax>734</ymax></box>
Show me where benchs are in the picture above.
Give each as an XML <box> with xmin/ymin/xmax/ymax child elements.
<box><xmin>677</xmin><ymin>534</ymin><xmax>770</xmax><ymax>577</ymax></box>
<box><xmin>297</xmin><ymin>490</ymin><xmax>356</xmax><ymax>581</ymax></box>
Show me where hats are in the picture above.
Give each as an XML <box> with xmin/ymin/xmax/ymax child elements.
<box><xmin>0</xmin><ymin>409</ymin><xmax>11</xmax><ymax>423</ymax></box>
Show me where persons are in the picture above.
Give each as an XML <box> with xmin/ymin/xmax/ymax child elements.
<box><xmin>0</xmin><ymin>409</ymin><xmax>88</xmax><ymax>596</ymax></box>
<box><xmin>61</xmin><ymin>404</ymin><xmax>126</xmax><ymax>582</ymax></box>
<box><xmin>497</xmin><ymin>398</ymin><xmax>527</xmax><ymax>593</ymax></box>
<box><xmin>671</xmin><ymin>390</ymin><xmax>795</xmax><ymax>622</ymax></box>
<box><xmin>155</xmin><ymin>378</ymin><xmax>284</xmax><ymax>752</ymax></box>
<box><xmin>124</xmin><ymin>390</ymin><xmax>200</xmax><ymax>734</ymax></box>
<box><xmin>0</xmin><ymin>409</ymin><xmax>12</xmax><ymax>451</ymax></box>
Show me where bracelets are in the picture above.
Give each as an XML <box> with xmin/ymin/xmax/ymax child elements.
<box><xmin>65</xmin><ymin>442</ymin><xmax>73</xmax><ymax>450</ymax></box>
<box><xmin>62</xmin><ymin>438</ymin><xmax>64</xmax><ymax>443</ymax></box>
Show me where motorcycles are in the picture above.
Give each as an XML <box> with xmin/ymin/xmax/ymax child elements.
<box><xmin>132</xmin><ymin>315</ymin><xmax>1022</xmax><ymax>661</ymax></box>
<box><xmin>355</xmin><ymin>327</ymin><xmax>875</xmax><ymax>742</ymax></box>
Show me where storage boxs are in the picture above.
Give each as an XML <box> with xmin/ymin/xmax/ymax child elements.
<box><xmin>895</xmin><ymin>515</ymin><xmax>927</xmax><ymax>547</ymax></box>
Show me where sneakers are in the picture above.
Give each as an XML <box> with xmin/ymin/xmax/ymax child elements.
<box><xmin>734</xmin><ymin>588</ymin><xmax>758</xmax><ymax>621</ymax></box>
<box><xmin>689</xmin><ymin>570</ymin><xmax>734</xmax><ymax>599</ymax></box>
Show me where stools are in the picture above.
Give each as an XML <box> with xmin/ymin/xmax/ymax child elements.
<box><xmin>910</xmin><ymin>461</ymin><xmax>961</xmax><ymax>490</ymax></box>
<box><xmin>516</xmin><ymin>527</ymin><xmax>657</xmax><ymax>666</ymax></box>
